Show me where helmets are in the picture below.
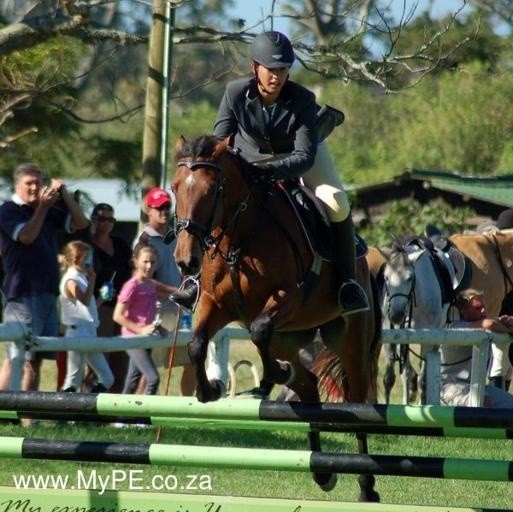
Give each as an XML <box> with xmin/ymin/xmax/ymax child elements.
<box><xmin>252</xmin><ymin>31</ymin><xmax>294</xmax><ymax>68</ymax></box>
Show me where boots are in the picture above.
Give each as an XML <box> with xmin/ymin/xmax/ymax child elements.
<box><xmin>168</xmin><ymin>279</ymin><xmax>200</xmax><ymax>309</ymax></box>
<box><xmin>331</xmin><ymin>210</ymin><xmax>368</xmax><ymax>328</ymax></box>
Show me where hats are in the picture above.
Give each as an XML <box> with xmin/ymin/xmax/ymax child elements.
<box><xmin>146</xmin><ymin>189</ymin><xmax>171</xmax><ymax>208</ymax></box>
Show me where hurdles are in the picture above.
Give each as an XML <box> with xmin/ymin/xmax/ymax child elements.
<box><xmin>0</xmin><ymin>391</ymin><xmax>513</xmax><ymax>512</ymax></box>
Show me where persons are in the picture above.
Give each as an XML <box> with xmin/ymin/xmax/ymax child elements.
<box><xmin>415</xmin><ymin>285</ymin><xmax>513</xmax><ymax>409</ymax></box>
<box><xmin>0</xmin><ymin>161</ymin><xmax>201</xmax><ymax>431</ymax></box>
<box><xmin>165</xmin><ymin>30</ymin><xmax>372</xmax><ymax>320</ymax></box>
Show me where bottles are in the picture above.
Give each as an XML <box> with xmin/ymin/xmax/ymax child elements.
<box><xmin>183</xmin><ymin>311</ymin><xmax>191</xmax><ymax>329</ymax></box>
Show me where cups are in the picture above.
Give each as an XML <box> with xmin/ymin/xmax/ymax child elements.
<box><xmin>102</xmin><ymin>283</ymin><xmax>114</xmax><ymax>301</ymax></box>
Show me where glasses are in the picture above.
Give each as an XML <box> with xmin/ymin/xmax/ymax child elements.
<box><xmin>96</xmin><ymin>215</ymin><xmax>116</xmax><ymax>222</ymax></box>
